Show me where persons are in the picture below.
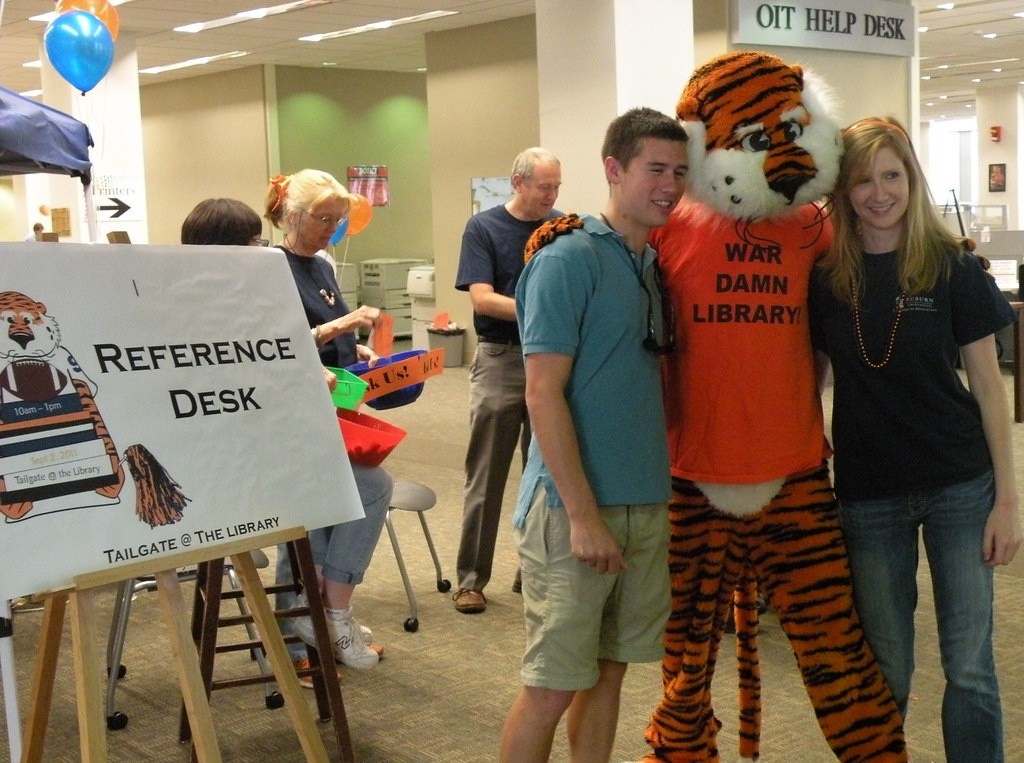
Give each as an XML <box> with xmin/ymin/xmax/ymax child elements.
<box><xmin>452</xmin><ymin>148</ymin><xmax>564</xmax><ymax>611</ymax></box>
<box><xmin>812</xmin><ymin>117</ymin><xmax>1024</xmax><ymax>762</ymax></box>
<box><xmin>498</xmin><ymin>109</ymin><xmax>689</xmax><ymax>763</ymax></box>
<box><xmin>26</xmin><ymin>224</ymin><xmax>45</xmax><ymax>241</ymax></box>
<box><xmin>183</xmin><ymin>167</ymin><xmax>393</xmax><ymax>688</ymax></box>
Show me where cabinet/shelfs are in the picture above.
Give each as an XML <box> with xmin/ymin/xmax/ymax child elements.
<box><xmin>407</xmin><ymin>263</ymin><xmax>435</xmax><ymax>353</ymax></box>
<box><xmin>336</xmin><ymin>262</ymin><xmax>360</xmax><ymax>344</ymax></box>
<box><xmin>359</xmin><ymin>257</ymin><xmax>434</xmax><ymax>343</ymax></box>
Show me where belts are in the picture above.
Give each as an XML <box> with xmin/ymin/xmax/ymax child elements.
<box><xmin>478</xmin><ymin>334</ymin><xmax>521</xmax><ymax>345</ymax></box>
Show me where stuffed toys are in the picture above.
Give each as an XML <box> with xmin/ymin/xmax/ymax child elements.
<box><xmin>522</xmin><ymin>50</ymin><xmax>990</xmax><ymax>762</ymax></box>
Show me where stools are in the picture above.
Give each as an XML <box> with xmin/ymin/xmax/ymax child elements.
<box><xmin>177</xmin><ymin>531</ymin><xmax>355</xmax><ymax>763</ymax></box>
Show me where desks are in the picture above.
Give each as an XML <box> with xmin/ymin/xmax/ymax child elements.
<box><xmin>1009</xmin><ymin>301</ymin><xmax>1024</xmax><ymax>424</ymax></box>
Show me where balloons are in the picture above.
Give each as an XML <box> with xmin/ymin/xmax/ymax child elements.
<box><xmin>53</xmin><ymin>0</ymin><xmax>118</xmax><ymax>42</ymax></box>
<box><xmin>44</xmin><ymin>9</ymin><xmax>113</xmax><ymax>95</ymax></box>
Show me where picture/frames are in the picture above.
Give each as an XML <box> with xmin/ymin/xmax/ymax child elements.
<box><xmin>989</xmin><ymin>164</ymin><xmax>1006</xmax><ymax>192</ymax></box>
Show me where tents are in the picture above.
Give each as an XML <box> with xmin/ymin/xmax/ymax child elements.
<box><xmin>0</xmin><ymin>86</ymin><xmax>97</xmax><ymax>241</ymax></box>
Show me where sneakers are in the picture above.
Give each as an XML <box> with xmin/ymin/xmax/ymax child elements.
<box><xmin>293</xmin><ymin>600</ymin><xmax>379</xmax><ymax>670</ymax></box>
<box><xmin>293</xmin><ymin>659</ymin><xmax>342</xmax><ymax>688</ymax></box>
<box><xmin>288</xmin><ymin>594</ymin><xmax>373</xmax><ymax>647</ymax></box>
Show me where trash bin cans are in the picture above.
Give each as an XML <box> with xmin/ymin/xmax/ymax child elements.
<box><xmin>426</xmin><ymin>327</ymin><xmax>465</xmax><ymax>367</ymax></box>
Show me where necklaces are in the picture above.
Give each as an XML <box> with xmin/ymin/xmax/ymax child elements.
<box><xmin>286</xmin><ymin>237</ymin><xmax>335</xmax><ymax>306</ymax></box>
<box><xmin>850</xmin><ymin>273</ymin><xmax>905</xmax><ymax>369</ymax></box>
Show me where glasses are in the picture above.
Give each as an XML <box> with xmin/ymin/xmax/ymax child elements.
<box><xmin>299</xmin><ymin>207</ymin><xmax>348</xmax><ymax>226</ymax></box>
<box><xmin>642</xmin><ymin>303</ymin><xmax>675</xmax><ymax>353</ymax></box>
<box><xmin>252</xmin><ymin>238</ymin><xmax>269</xmax><ymax>247</ymax></box>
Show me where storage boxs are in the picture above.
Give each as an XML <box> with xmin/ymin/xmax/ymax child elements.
<box><xmin>323</xmin><ymin>364</ymin><xmax>369</xmax><ymax>413</ymax></box>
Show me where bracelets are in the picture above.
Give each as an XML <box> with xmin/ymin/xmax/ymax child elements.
<box><xmin>316</xmin><ymin>326</ymin><xmax>322</xmax><ymax>344</ymax></box>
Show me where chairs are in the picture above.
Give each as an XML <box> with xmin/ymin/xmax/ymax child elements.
<box><xmin>385</xmin><ymin>482</ymin><xmax>453</xmax><ymax>637</ymax></box>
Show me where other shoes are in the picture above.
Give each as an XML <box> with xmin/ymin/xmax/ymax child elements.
<box><xmin>512</xmin><ymin>581</ymin><xmax>521</xmax><ymax>592</ymax></box>
<box><xmin>456</xmin><ymin>580</ymin><xmax>487</xmax><ymax>613</ymax></box>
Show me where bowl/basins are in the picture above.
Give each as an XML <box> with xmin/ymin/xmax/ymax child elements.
<box><xmin>336</xmin><ymin>405</ymin><xmax>407</xmax><ymax>468</ymax></box>
<box><xmin>345</xmin><ymin>350</ymin><xmax>429</xmax><ymax>410</ymax></box>
